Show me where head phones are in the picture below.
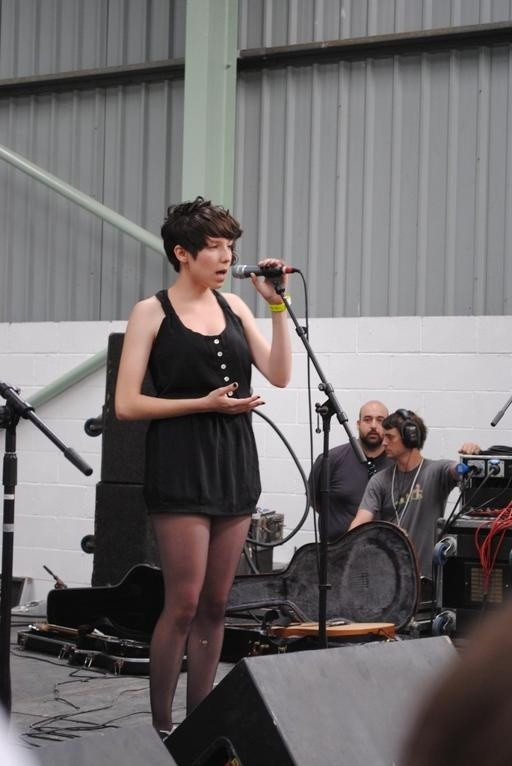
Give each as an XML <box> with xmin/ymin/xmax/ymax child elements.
<box><xmin>397</xmin><ymin>407</ymin><xmax>423</xmax><ymax>450</ymax></box>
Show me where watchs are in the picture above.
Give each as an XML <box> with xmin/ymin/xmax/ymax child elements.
<box><xmin>268</xmin><ymin>295</ymin><xmax>293</xmax><ymax>312</ymax></box>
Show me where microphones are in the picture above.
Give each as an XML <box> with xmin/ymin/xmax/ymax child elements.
<box><xmin>230</xmin><ymin>262</ymin><xmax>300</xmax><ymax>282</ymax></box>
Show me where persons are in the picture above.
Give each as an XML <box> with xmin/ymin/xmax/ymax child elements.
<box><xmin>115</xmin><ymin>196</ymin><xmax>295</xmax><ymax>746</ymax></box>
<box><xmin>305</xmin><ymin>400</ymin><xmax>395</xmax><ymax>547</ymax></box>
<box><xmin>346</xmin><ymin>408</ymin><xmax>484</xmax><ymax>610</ymax></box>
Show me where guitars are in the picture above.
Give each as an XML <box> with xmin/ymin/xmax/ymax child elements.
<box><xmin>33</xmin><ymin>622</ymin><xmax>151</xmax><ymax>658</ymax></box>
<box><xmin>225</xmin><ymin>619</ymin><xmax>396</xmax><ymax>641</ymax></box>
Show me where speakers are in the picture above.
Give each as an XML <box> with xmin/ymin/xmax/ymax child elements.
<box><xmin>164</xmin><ymin>634</ymin><xmax>458</xmax><ymax>766</ymax></box>
<box><xmin>91</xmin><ymin>481</ymin><xmax>163</xmax><ymax>588</ymax></box>
<box><xmin>99</xmin><ymin>332</ymin><xmax>157</xmax><ymax>481</ymax></box>
